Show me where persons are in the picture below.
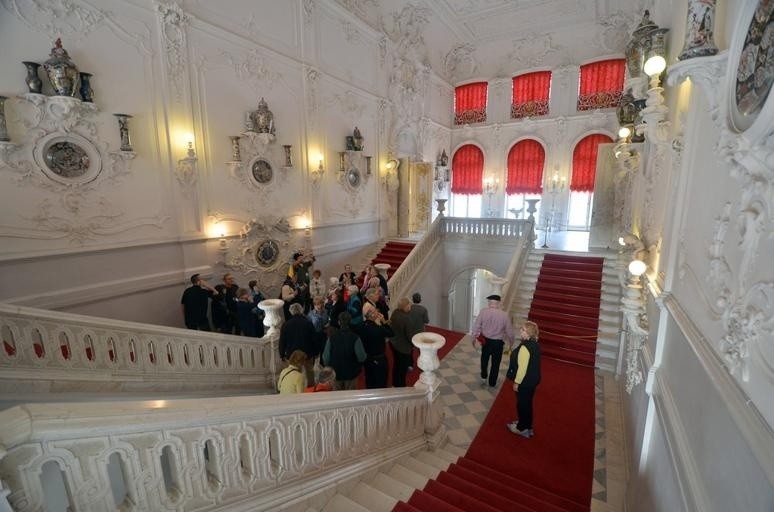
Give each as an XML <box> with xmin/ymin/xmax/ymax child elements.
<box><xmin>504</xmin><ymin>320</ymin><xmax>542</xmax><ymax>438</ymax></box>
<box><xmin>469</xmin><ymin>293</ymin><xmax>515</xmax><ymax>389</ymax></box>
<box><xmin>177</xmin><ymin>250</ymin><xmax>431</xmax><ymax>395</ymax></box>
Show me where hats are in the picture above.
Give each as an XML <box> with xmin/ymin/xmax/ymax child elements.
<box><xmin>487</xmin><ymin>295</ymin><xmax>500</xmax><ymax>300</ymax></box>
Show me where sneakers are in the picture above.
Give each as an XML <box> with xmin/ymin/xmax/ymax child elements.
<box><xmin>481</xmin><ymin>378</ymin><xmax>487</xmax><ymax>384</ymax></box>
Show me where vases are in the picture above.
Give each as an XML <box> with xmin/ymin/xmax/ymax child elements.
<box><xmin>23</xmin><ymin>61</ymin><xmax>43</xmax><ymax>93</ymax></box>
<box><xmin>112</xmin><ymin>112</ymin><xmax>135</xmax><ymax>151</ymax></box>
<box><xmin>80</xmin><ymin>71</ymin><xmax>96</xmax><ymax>103</ymax></box>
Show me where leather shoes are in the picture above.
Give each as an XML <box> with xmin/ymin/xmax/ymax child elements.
<box><xmin>506</xmin><ymin>423</ymin><xmax>528</xmax><ymax>436</ymax></box>
<box><xmin>513</xmin><ymin>421</ymin><xmax>534</xmax><ymax>436</ymax></box>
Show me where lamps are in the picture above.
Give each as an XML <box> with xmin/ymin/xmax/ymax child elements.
<box><xmin>621</xmin><ymin>260</ymin><xmax>649</xmax><ymax>340</ymax></box>
<box><xmin>636</xmin><ymin>54</ymin><xmax>671</xmax><ymax>148</ymax></box>
